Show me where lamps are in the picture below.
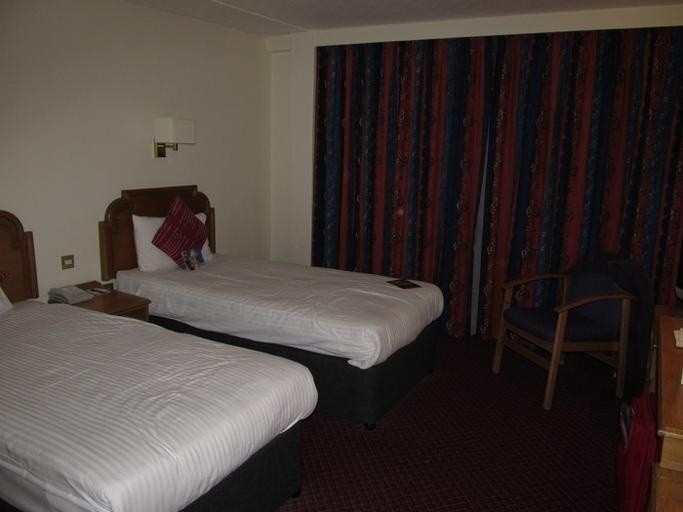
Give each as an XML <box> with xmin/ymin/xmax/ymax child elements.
<box><xmin>152</xmin><ymin>118</ymin><xmax>196</xmax><ymax>158</ymax></box>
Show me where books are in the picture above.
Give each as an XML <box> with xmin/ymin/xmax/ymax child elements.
<box><xmin>387</xmin><ymin>277</ymin><xmax>421</xmax><ymax>291</ymax></box>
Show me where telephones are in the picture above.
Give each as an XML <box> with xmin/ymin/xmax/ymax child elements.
<box><xmin>47</xmin><ymin>286</ymin><xmax>95</xmax><ymax>305</ymax></box>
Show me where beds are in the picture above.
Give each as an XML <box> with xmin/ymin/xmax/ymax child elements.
<box><xmin>0</xmin><ymin>210</ymin><xmax>320</xmax><ymax>512</ymax></box>
<box><xmin>98</xmin><ymin>185</ymin><xmax>450</xmax><ymax>432</ymax></box>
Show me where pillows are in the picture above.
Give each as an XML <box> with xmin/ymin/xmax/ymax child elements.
<box><xmin>151</xmin><ymin>194</ymin><xmax>210</xmax><ymax>271</ymax></box>
<box><xmin>132</xmin><ymin>212</ymin><xmax>213</xmax><ymax>273</ymax></box>
<box><xmin>0</xmin><ymin>286</ymin><xmax>13</xmax><ymax>315</ymax></box>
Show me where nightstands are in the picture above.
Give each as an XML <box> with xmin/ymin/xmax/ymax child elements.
<box><xmin>47</xmin><ymin>280</ymin><xmax>151</xmax><ymax>323</ymax></box>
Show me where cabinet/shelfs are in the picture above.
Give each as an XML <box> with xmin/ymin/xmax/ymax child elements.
<box><xmin>616</xmin><ymin>304</ymin><xmax>682</xmax><ymax>512</ymax></box>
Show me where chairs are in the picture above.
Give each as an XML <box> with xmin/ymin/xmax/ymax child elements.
<box><xmin>491</xmin><ymin>260</ymin><xmax>636</xmax><ymax>410</ymax></box>
